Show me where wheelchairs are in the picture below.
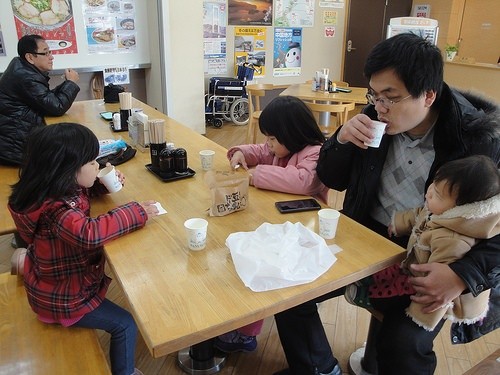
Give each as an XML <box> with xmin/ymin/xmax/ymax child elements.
<box><xmin>205</xmin><ymin>61</ymin><xmax>260</xmax><ymax>128</ymax></box>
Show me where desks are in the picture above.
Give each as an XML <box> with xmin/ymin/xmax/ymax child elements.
<box><xmin>43</xmin><ymin>96</ymin><xmax>411</xmax><ymax>360</ymax></box>
<box><xmin>279</xmin><ymin>82</ymin><xmax>369</xmax><ymax>130</ymax></box>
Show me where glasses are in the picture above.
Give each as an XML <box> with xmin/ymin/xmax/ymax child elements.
<box><xmin>33</xmin><ymin>50</ymin><xmax>52</xmax><ymax>57</ymax></box>
<box><xmin>366</xmin><ymin>89</ymin><xmax>412</xmax><ymax>109</ymax></box>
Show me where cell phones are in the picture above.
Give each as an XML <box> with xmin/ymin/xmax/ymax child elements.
<box><xmin>100</xmin><ymin>111</ymin><xmax>113</xmax><ymax>120</ymax></box>
<box><xmin>275</xmin><ymin>199</ymin><xmax>321</xmax><ymax>213</ymax></box>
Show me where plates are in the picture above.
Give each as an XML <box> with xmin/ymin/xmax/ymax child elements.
<box><xmin>91</xmin><ymin>27</ymin><xmax>114</xmax><ymax>43</ymax></box>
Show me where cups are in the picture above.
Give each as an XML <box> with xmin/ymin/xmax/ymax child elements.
<box><xmin>150</xmin><ymin>143</ymin><xmax>166</xmax><ymax>163</ymax></box>
<box><xmin>363</xmin><ymin>120</ymin><xmax>387</xmax><ymax>148</ymax></box>
<box><xmin>199</xmin><ymin>150</ymin><xmax>215</xmax><ymax>170</ymax></box>
<box><xmin>184</xmin><ymin>218</ymin><xmax>208</xmax><ymax>250</ymax></box>
<box><xmin>317</xmin><ymin>209</ymin><xmax>340</xmax><ymax>239</ymax></box>
<box><xmin>96</xmin><ymin>165</ymin><xmax>122</xmax><ymax>193</ymax></box>
<box><xmin>120</xmin><ymin>109</ymin><xmax>131</xmax><ymax>130</ymax></box>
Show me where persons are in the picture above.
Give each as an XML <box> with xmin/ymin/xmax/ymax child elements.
<box><xmin>0</xmin><ymin>35</ymin><xmax>80</xmax><ymax>248</ymax></box>
<box><xmin>216</xmin><ymin>96</ymin><xmax>333</xmax><ymax>354</ymax></box>
<box><xmin>7</xmin><ymin>122</ymin><xmax>159</xmax><ymax>375</ymax></box>
<box><xmin>276</xmin><ymin>30</ymin><xmax>500</xmax><ymax>375</ymax></box>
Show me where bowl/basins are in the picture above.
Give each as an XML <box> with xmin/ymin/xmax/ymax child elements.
<box><xmin>120</xmin><ymin>18</ymin><xmax>134</xmax><ymax>30</ymax></box>
<box><xmin>119</xmin><ymin>36</ymin><xmax>136</xmax><ymax>47</ymax></box>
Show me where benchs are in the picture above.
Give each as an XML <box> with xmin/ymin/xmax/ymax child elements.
<box><xmin>0</xmin><ymin>265</ymin><xmax>112</xmax><ymax>375</ymax></box>
<box><xmin>0</xmin><ymin>166</ymin><xmax>19</xmax><ymax>236</ymax></box>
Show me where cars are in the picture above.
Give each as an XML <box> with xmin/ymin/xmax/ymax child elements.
<box><xmin>249</xmin><ymin>51</ymin><xmax>265</xmax><ymax>66</ymax></box>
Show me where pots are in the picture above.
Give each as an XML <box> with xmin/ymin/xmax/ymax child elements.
<box><xmin>11</xmin><ymin>0</ymin><xmax>74</xmax><ymax>30</ymax></box>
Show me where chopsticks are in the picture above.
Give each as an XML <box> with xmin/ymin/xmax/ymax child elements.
<box><xmin>118</xmin><ymin>92</ymin><xmax>132</xmax><ymax>110</ymax></box>
<box><xmin>147</xmin><ymin>119</ymin><xmax>166</xmax><ymax>143</ymax></box>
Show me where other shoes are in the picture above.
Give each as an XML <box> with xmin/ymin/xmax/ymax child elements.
<box><xmin>344</xmin><ymin>284</ymin><xmax>372</xmax><ymax>308</ymax></box>
<box><xmin>355</xmin><ymin>279</ymin><xmax>366</xmax><ymax>286</ymax></box>
<box><xmin>223</xmin><ymin>335</ymin><xmax>257</xmax><ymax>351</ymax></box>
<box><xmin>272</xmin><ymin>358</ymin><xmax>341</xmax><ymax>375</ymax></box>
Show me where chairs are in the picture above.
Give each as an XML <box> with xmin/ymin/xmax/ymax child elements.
<box><xmin>305</xmin><ymin>78</ymin><xmax>349</xmax><ymax>87</ymax></box>
<box><xmin>305</xmin><ymin>100</ymin><xmax>357</xmax><ymax>142</ymax></box>
<box><xmin>242</xmin><ymin>83</ymin><xmax>273</xmax><ymax>145</ymax></box>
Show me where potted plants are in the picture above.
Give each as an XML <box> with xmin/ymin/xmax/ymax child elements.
<box><xmin>444</xmin><ymin>41</ymin><xmax>459</xmax><ymax>63</ymax></box>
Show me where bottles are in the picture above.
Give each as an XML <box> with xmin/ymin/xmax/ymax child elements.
<box><xmin>158</xmin><ymin>143</ymin><xmax>188</xmax><ymax>178</ymax></box>
<box><xmin>319</xmin><ymin>74</ymin><xmax>336</xmax><ymax>92</ymax></box>
